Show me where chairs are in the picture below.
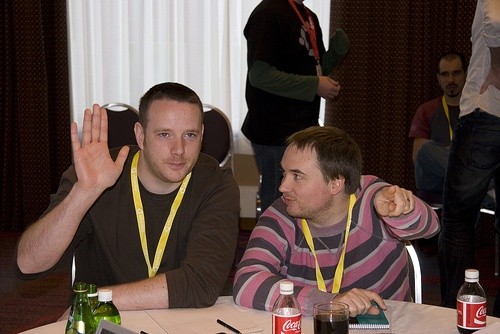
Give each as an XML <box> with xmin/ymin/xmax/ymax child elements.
<box><xmin>100</xmin><ymin>103</ymin><xmax>140</xmax><ymax>148</ymax></box>
<box><xmin>200</xmin><ymin>104</ymin><xmax>236</xmax><ymax>176</ymax></box>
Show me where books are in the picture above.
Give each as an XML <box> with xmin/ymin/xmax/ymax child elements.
<box><xmin>348</xmin><ymin>302</ymin><xmax>390</xmax><ymax>329</ymax></box>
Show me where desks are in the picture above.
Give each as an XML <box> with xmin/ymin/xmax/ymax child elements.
<box><xmin>17</xmin><ymin>294</ymin><xmax>500</xmax><ymax>334</ymax></box>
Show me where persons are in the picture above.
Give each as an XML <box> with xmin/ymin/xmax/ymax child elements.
<box><xmin>409</xmin><ymin>52</ymin><xmax>469</xmax><ymax>204</ymax></box>
<box><xmin>241</xmin><ymin>0</ymin><xmax>340</xmax><ymax>210</ymax></box>
<box><xmin>11</xmin><ymin>82</ymin><xmax>240</xmax><ymax>322</ymax></box>
<box><xmin>437</xmin><ymin>0</ymin><xmax>500</xmax><ymax>317</ymax></box>
<box><xmin>233</xmin><ymin>126</ymin><xmax>441</xmax><ymax>316</ymax></box>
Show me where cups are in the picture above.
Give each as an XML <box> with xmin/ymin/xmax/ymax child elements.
<box><xmin>313</xmin><ymin>301</ymin><xmax>350</xmax><ymax>334</ymax></box>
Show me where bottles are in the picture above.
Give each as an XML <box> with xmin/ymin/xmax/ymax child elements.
<box><xmin>456</xmin><ymin>269</ymin><xmax>486</xmax><ymax>334</ymax></box>
<box><xmin>65</xmin><ymin>281</ymin><xmax>121</xmax><ymax>334</ymax></box>
<box><xmin>272</xmin><ymin>279</ymin><xmax>302</xmax><ymax>334</ymax></box>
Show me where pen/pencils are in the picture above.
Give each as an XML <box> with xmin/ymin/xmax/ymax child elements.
<box><xmin>217</xmin><ymin>319</ymin><xmax>242</xmax><ymax>334</ymax></box>
